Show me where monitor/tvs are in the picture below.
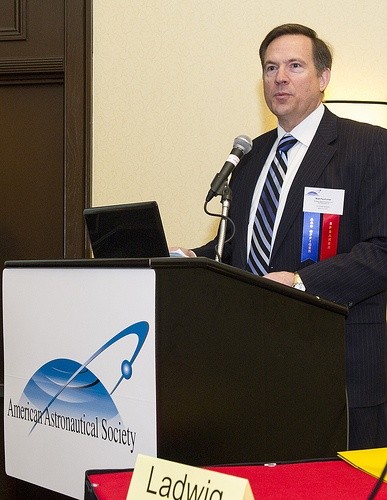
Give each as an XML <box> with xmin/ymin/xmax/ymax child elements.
<box><xmin>85</xmin><ymin>201</ymin><xmax>170</xmax><ymax>259</ymax></box>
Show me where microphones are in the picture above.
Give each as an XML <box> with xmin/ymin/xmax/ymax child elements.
<box><xmin>206</xmin><ymin>135</ymin><xmax>253</xmax><ymax>202</ymax></box>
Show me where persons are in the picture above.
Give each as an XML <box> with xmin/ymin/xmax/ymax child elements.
<box><xmin>170</xmin><ymin>20</ymin><xmax>387</xmax><ymax>450</ymax></box>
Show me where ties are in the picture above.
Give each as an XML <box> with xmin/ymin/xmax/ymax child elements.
<box><xmin>245</xmin><ymin>132</ymin><xmax>299</xmax><ymax>278</ymax></box>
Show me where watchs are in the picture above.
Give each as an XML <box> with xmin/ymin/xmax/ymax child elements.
<box><xmin>292</xmin><ymin>272</ymin><xmax>307</xmax><ymax>294</ymax></box>
